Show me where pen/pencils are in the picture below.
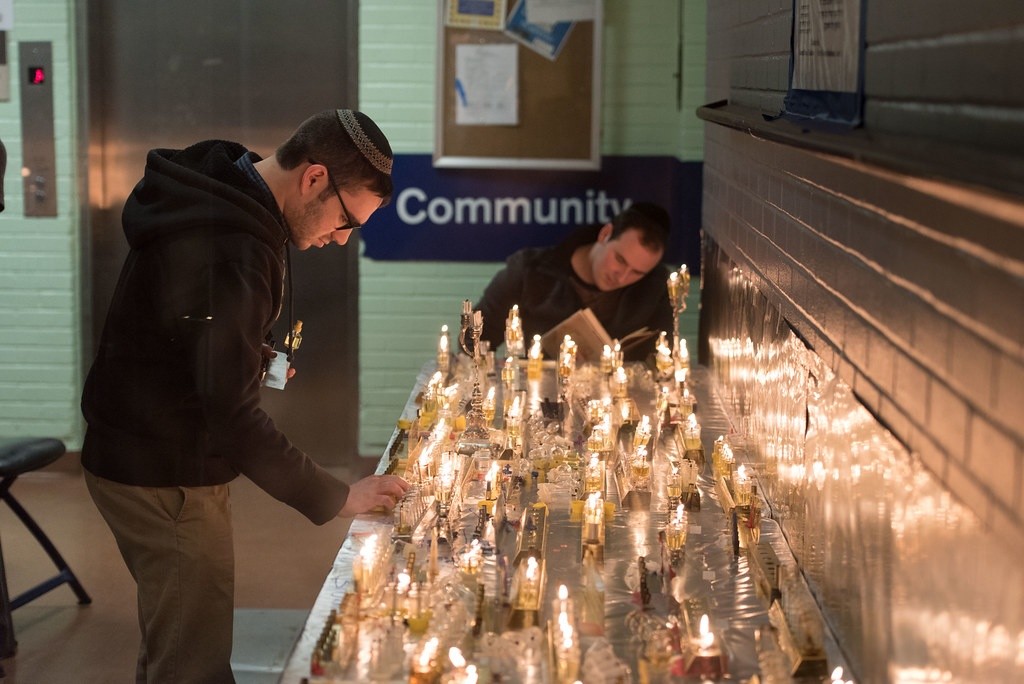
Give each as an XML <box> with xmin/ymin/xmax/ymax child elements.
<box><xmin>260</xmin><ymin>341</ymin><xmax>277</xmax><ymax>383</ymax></box>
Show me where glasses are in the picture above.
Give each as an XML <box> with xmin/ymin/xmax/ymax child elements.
<box><xmin>307</xmin><ymin>157</ymin><xmax>362</xmax><ymax>231</ymax></box>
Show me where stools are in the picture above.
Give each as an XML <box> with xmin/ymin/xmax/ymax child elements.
<box><xmin>1</xmin><ymin>435</ymin><xmax>95</xmax><ymax>657</ymax></box>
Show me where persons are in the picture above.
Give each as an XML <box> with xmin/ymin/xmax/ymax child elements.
<box><xmin>80</xmin><ymin>105</ymin><xmax>409</xmax><ymax>683</ymax></box>
<box><xmin>454</xmin><ymin>201</ymin><xmax>675</xmax><ymax>364</ymax></box>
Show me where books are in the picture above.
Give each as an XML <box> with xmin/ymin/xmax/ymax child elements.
<box><xmin>538</xmin><ymin>307</ymin><xmax>661</xmax><ymax>364</ymax></box>
